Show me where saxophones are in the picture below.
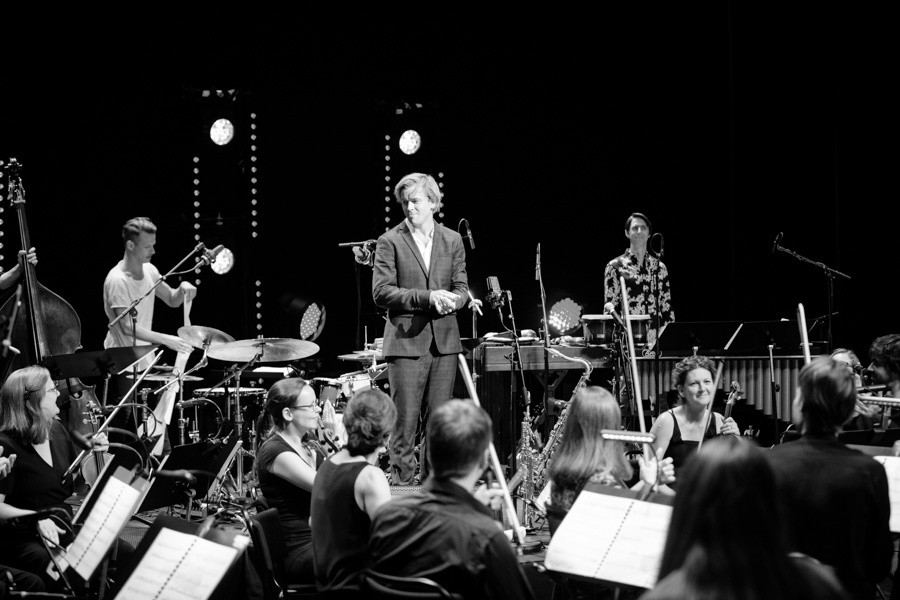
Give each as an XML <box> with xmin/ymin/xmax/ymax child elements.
<box><xmin>511</xmin><ymin>347</ymin><xmax>595</xmax><ymax>533</ymax></box>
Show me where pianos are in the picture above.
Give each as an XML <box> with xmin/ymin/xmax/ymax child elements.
<box><xmin>479</xmin><ymin>340</ymin><xmax>616</xmax><ymax>480</ymax></box>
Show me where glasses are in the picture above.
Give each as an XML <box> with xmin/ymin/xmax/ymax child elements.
<box><xmin>44</xmin><ymin>381</ymin><xmax>59</xmax><ymax>393</ymax></box>
<box><xmin>293</xmin><ymin>399</ymin><xmax>318</xmax><ymax>409</ymax></box>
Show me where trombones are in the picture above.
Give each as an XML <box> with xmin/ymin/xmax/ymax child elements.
<box><xmin>796</xmin><ymin>302</ymin><xmax>812</xmax><ymax>364</ymax></box>
<box><xmin>456</xmin><ymin>352</ymin><xmax>528</xmax><ymax>552</ymax></box>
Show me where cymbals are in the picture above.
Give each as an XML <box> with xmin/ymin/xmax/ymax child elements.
<box><xmin>336</xmin><ymin>350</ymin><xmax>385</xmax><ymax>361</ymax></box>
<box><xmin>125</xmin><ymin>365</ymin><xmax>205</xmax><ymax>382</ymax></box>
<box><xmin>177</xmin><ymin>324</ymin><xmax>236</xmax><ymax>351</ymax></box>
<box><xmin>205</xmin><ymin>336</ymin><xmax>321</xmax><ymax>363</ymax></box>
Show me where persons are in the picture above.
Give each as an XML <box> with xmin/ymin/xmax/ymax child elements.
<box><xmin>829</xmin><ymin>348</ymin><xmax>862</xmax><ymax>388</ymax></box>
<box><xmin>649</xmin><ymin>355</ymin><xmax>740</xmax><ymax>496</ymax></box>
<box><xmin>0</xmin><ymin>446</ymin><xmax>17</xmax><ymax>479</ymax></box>
<box><xmin>640</xmin><ymin>434</ymin><xmax>860</xmax><ymax>600</ymax></box>
<box><xmin>0</xmin><ymin>247</ymin><xmax>38</xmax><ymax>292</ymax></box>
<box><xmin>373</xmin><ymin>172</ymin><xmax>469</xmax><ymax>486</ymax></box>
<box><xmin>103</xmin><ymin>216</ymin><xmax>197</xmax><ymax>419</ymax></box>
<box><xmin>547</xmin><ymin>386</ymin><xmax>675</xmax><ymax>513</ymax></box>
<box><xmin>603</xmin><ymin>213</ymin><xmax>675</xmax><ymax>357</ymax></box>
<box><xmin>256</xmin><ymin>378</ymin><xmax>329</xmax><ymax>591</ymax></box>
<box><xmin>867</xmin><ymin>334</ymin><xmax>900</xmax><ymax>429</ymax></box>
<box><xmin>368</xmin><ymin>399</ymin><xmax>538</xmax><ymax>600</ymax></box>
<box><xmin>311</xmin><ymin>387</ymin><xmax>398</xmax><ymax>589</ymax></box>
<box><xmin>0</xmin><ymin>365</ymin><xmax>136</xmax><ymax>600</ymax></box>
<box><xmin>767</xmin><ymin>355</ymin><xmax>891</xmax><ymax>599</ymax></box>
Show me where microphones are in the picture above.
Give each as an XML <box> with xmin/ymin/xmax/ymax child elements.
<box><xmin>773</xmin><ymin>232</ymin><xmax>783</xmax><ymax>244</ymax></box>
<box><xmin>352</xmin><ymin>247</ymin><xmax>372</xmax><ymax>266</ymax></box>
<box><xmin>536</xmin><ymin>250</ymin><xmax>540</xmax><ymax>279</ymax></box>
<box><xmin>604</xmin><ymin>302</ymin><xmax>626</xmax><ymax>330</ymax></box>
<box><xmin>487</xmin><ymin>276</ymin><xmax>505</xmax><ymax>308</ymax></box>
<box><xmin>290</xmin><ymin>365</ymin><xmax>305</xmax><ymax>376</ymax></box>
<box><xmin>195</xmin><ymin>244</ymin><xmax>224</xmax><ymax>268</ymax></box>
<box><xmin>465</xmin><ymin>220</ymin><xmax>475</xmax><ymax>249</ymax></box>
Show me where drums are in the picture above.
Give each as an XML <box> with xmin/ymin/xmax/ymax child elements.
<box><xmin>194</xmin><ymin>386</ymin><xmax>267</xmax><ymax>455</ymax></box>
<box><xmin>621</xmin><ymin>315</ymin><xmax>661</xmax><ymax>347</ymax></box>
<box><xmin>580</xmin><ymin>313</ymin><xmax>618</xmax><ymax>346</ymax></box>
<box><xmin>311</xmin><ymin>376</ymin><xmax>343</xmax><ymax>408</ymax></box>
<box><xmin>338</xmin><ymin>369</ymin><xmax>383</xmax><ymax>398</ymax></box>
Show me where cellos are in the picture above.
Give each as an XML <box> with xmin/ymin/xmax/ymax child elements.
<box><xmin>0</xmin><ymin>156</ymin><xmax>105</xmax><ymax>503</ymax></box>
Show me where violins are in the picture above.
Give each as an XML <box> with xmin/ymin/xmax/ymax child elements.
<box><xmin>79</xmin><ymin>398</ymin><xmax>114</xmax><ymax>492</ymax></box>
<box><xmin>716</xmin><ymin>380</ymin><xmax>745</xmax><ymax>444</ymax></box>
<box><xmin>319</xmin><ymin>427</ymin><xmax>342</xmax><ymax>454</ymax></box>
